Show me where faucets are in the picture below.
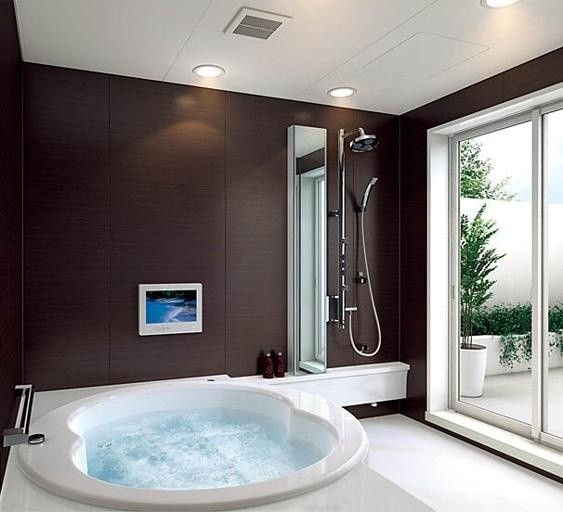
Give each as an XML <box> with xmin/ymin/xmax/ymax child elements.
<box><xmin>20</xmin><ymin>387</ymin><xmax>38</xmax><ymax>436</ymax></box>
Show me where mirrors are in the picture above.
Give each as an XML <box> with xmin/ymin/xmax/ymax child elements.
<box><xmin>292</xmin><ymin>124</ymin><xmax>327</xmax><ymax>375</ymax></box>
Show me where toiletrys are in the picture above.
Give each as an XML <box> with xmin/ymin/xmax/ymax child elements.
<box><xmin>262</xmin><ymin>352</ymin><xmax>273</xmax><ymax>378</ymax></box>
<box><xmin>275</xmin><ymin>352</ymin><xmax>284</xmax><ymax>376</ymax></box>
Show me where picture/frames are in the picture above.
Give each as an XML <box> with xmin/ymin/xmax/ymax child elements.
<box><xmin>138</xmin><ymin>283</ymin><xmax>204</xmax><ymax>336</ymax></box>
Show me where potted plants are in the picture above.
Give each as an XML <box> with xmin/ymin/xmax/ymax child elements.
<box><xmin>459</xmin><ymin>204</ymin><xmax>508</xmax><ymax>398</ymax></box>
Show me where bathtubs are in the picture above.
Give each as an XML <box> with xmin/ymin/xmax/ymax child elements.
<box><xmin>17</xmin><ymin>376</ymin><xmax>365</xmax><ymax>511</ymax></box>
